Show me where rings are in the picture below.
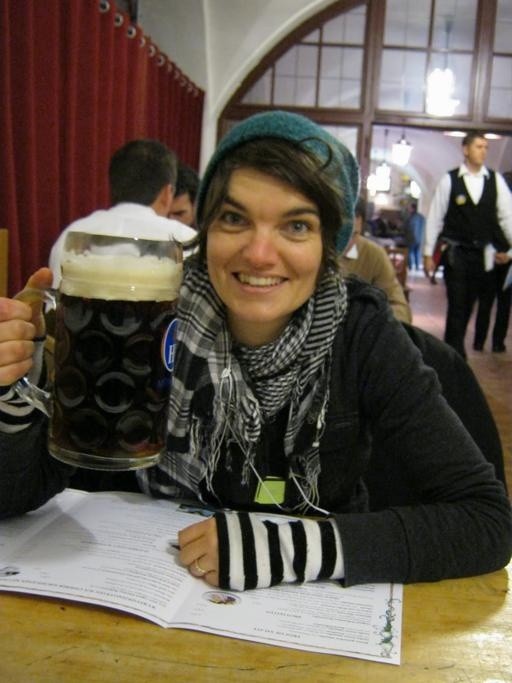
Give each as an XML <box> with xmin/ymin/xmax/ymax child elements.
<box><xmin>195</xmin><ymin>559</ymin><xmax>208</xmax><ymax>574</ymax></box>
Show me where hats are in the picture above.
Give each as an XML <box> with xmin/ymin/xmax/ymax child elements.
<box><xmin>197</xmin><ymin>110</ymin><xmax>357</xmax><ymax>257</ymax></box>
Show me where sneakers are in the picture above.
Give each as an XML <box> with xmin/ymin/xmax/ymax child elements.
<box><xmin>471</xmin><ymin>335</ymin><xmax>506</xmax><ymax>352</ymax></box>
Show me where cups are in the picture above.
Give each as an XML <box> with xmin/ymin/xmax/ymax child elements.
<box><xmin>9</xmin><ymin>274</ymin><xmax>185</xmax><ymax>473</ymax></box>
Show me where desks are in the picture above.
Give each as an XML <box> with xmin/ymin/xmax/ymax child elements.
<box><xmin>1</xmin><ymin>566</ymin><xmax>510</xmax><ymax>683</ymax></box>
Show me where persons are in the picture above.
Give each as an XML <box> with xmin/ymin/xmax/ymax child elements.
<box><xmin>0</xmin><ymin>110</ymin><xmax>512</xmax><ymax>592</ymax></box>
